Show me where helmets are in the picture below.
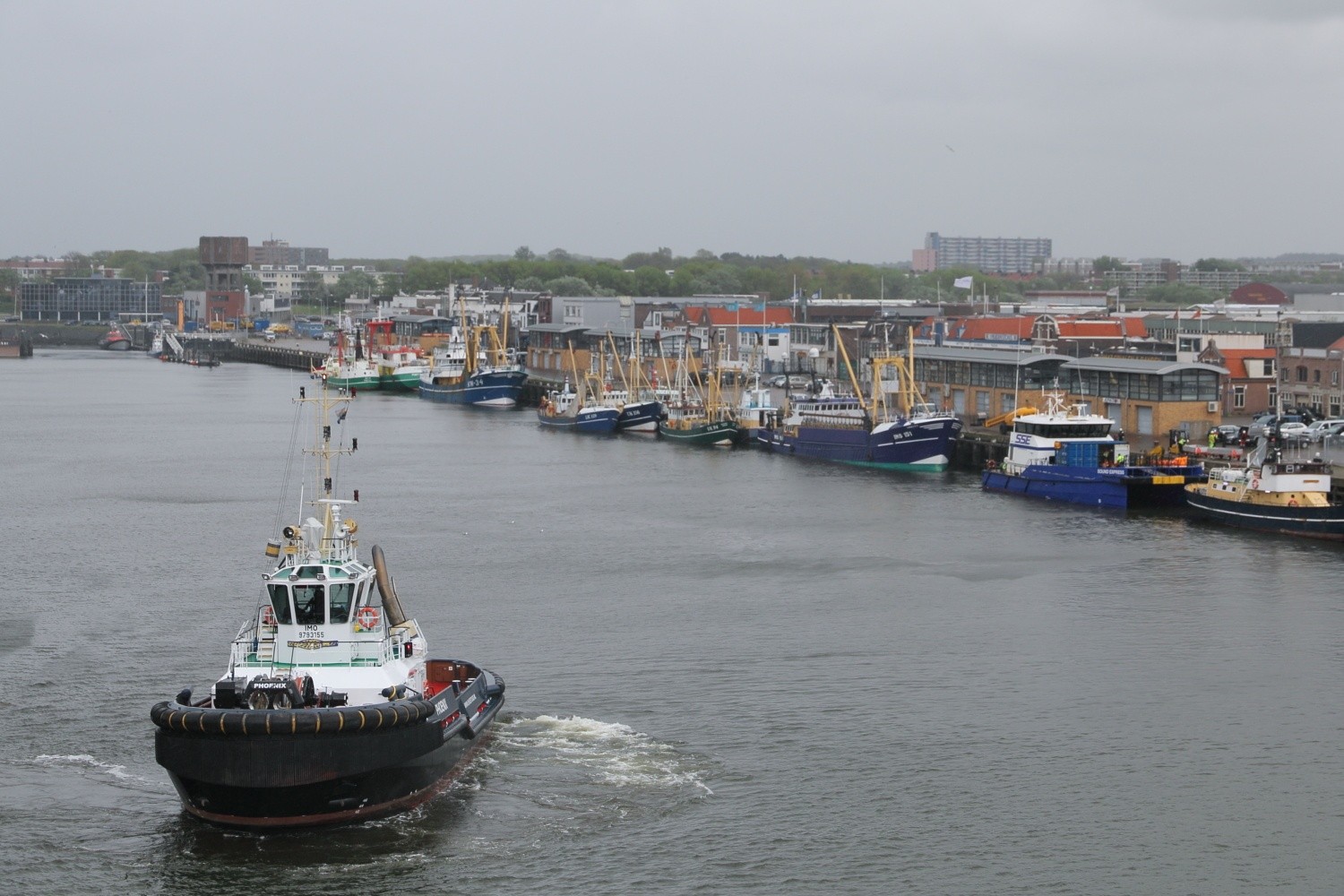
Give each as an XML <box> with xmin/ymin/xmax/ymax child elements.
<box><xmin>1243</xmin><ymin>430</ymin><xmax>1246</xmax><ymax>434</ymax></box>
<box><xmin>1120</xmin><ymin>428</ymin><xmax>1123</xmax><ymax>431</ymax></box>
<box><xmin>1211</xmin><ymin>431</ymin><xmax>1214</xmax><ymax>433</ymax></box>
<box><xmin>1214</xmin><ymin>430</ymin><xmax>1217</xmax><ymax>433</ymax></box>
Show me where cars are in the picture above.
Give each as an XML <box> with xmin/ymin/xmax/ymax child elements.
<box><xmin>762</xmin><ymin>375</ymin><xmax>786</xmax><ymax>387</ymax></box>
<box><xmin>775</xmin><ymin>376</ymin><xmax>809</xmax><ymax>390</ymax></box>
<box><xmin>738</xmin><ymin>370</ymin><xmax>761</xmax><ymax>384</ymax></box>
<box><xmin>1206</xmin><ymin>405</ymin><xmax>1344</xmax><ymax>451</ymax></box>
<box><xmin>4</xmin><ymin>315</ymin><xmax>21</xmax><ymax>323</ymax></box>
<box><xmin>64</xmin><ymin>319</ymin><xmax>109</xmax><ymax>327</ymax></box>
<box><xmin>808</xmin><ymin>379</ymin><xmax>834</xmax><ymax>393</ymax></box>
<box><xmin>914</xmin><ymin>402</ymin><xmax>937</xmax><ymax>416</ymax></box>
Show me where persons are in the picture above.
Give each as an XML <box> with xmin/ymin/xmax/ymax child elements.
<box><xmin>309</xmin><ymin>590</ymin><xmax>324</xmax><ymax>613</ymax></box>
<box><xmin>1118</xmin><ymin>428</ymin><xmax>1125</xmax><ymax>441</ymax></box>
<box><xmin>1209</xmin><ymin>430</ymin><xmax>1218</xmax><ymax>447</ymax></box>
<box><xmin>1239</xmin><ymin>430</ymin><xmax>1247</xmax><ymax>448</ymax></box>
<box><xmin>1117</xmin><ymin>454</ymin><xmax>1121</xmax><ymax>464</ymax></box>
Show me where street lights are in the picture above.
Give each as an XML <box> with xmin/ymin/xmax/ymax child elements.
<box><xmin>318</xmin><ymin>299</ymin><xmax>325</xmax><ymax>329</ymax></box>
<box><xmin>334</xmin><ymin>300</ymin><xmax>341</xmax><ymax>329</ymax></box>
<box><xmin>326</xmin><ymin>294</ymin><xmax>334</xmax><ymax>318</ymax></box>
<box><xmin>315</xmin><ymin>297</ymin><xmax>323</xmax><ymax>324</ymax></box>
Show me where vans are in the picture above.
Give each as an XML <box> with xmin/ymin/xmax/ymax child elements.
<box><xmin>263</xmin><ymin>330</ymin><xmax>276</xmax><ymax>343</ymax></box>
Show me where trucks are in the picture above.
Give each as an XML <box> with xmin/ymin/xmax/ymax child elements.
<box><xmin>183</xmin><ymin>314</ymin><xmax>335</xmax><ymax>340</ymax></box>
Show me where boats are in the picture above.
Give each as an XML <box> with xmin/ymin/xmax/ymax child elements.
<box><xmin>982</xmin><ymin>406</ymin><xmax>1039</xmax><ymax>435</ymax></box>
<box><xmin>307</xmin><ymin>282</ymin><xmax>780</xmax><ymax>448</ymax></box>
<box><xmin>1175</xmin><ymin>374</ymin><xmax>1344</xmax><ymax>543</ymax></box>
<box><xmin>979</xmin><ymin>315</ymin><xmax>1210</xmax><ymax>512</ymax></box>
<box><xmin>96</xmin><ymin>320</ymin><xmax>133</xmax><ymax>351</ymax></box>
<box><xmin>150</xmin><ymin>341</ymin><xmax>509</xmax><ymax>828</ymax></box>
<box><xmin>753</xmin><ymin>276</ymin><xmax>966</xmax><ymax>473</ymax></box>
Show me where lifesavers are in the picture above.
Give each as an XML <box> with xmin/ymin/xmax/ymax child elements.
<box><xmin>358</xmin><ymin>607</ymin><xmax>378</xmax><ymax>628</ymax></box>
<box><xmin>1288</xmin><ymin>499</ymin><xmax>1299</xmax><ymax>511</ymax></box>
<box><xmin>606</xmin><ymin>383</ymin><xmax>611</xmax><ymax>392</ymax></box>
<box><xmin>265</xmin><ymin>605</ymin><xmax>278</xmax><ymax>625</ymax></box>
<box><xmin>988</xmin><ymin>459</ymin><xmax>996</xmax><ymax>468</ymax></box>
<box><xmin>548</xmin><ymin>407</ymin><xmax>555</xmax><ymax>414</ymax></box>
<box><xmin>1252</xmin><ymin>479</ymin><xmax>1259</xmax><ymax>489</ymax></box>
